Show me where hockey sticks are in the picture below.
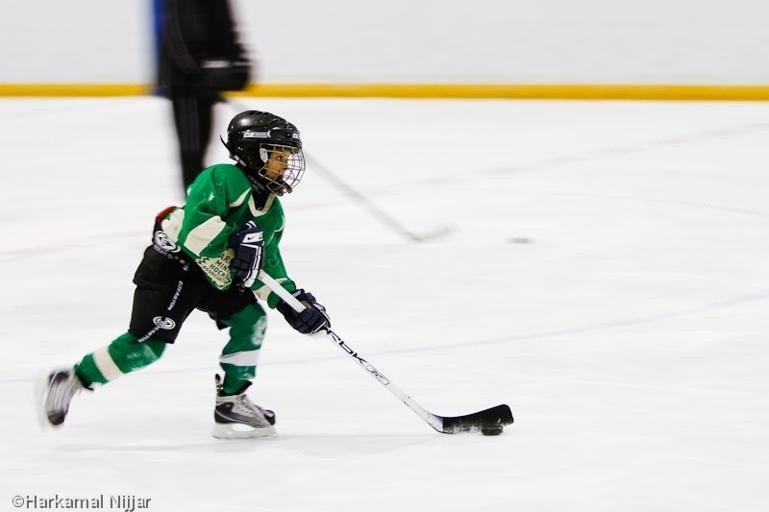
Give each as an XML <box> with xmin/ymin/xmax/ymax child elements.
<box><xmin>224</xmin><ymin>99</ymin><xmax>454</xmax><ymax>244</ymax></box>
<box><xmin>257</xmin><ymin>268</ymin><xmax>514</xmax><ymax>434</ymax></box>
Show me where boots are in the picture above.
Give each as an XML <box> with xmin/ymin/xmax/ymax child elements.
<box><xmin>213</xmin><ymin>374</ymin><xmax>277</xmax><ymax>428</ymax></box>
<box><xmin>46</xmin><ymin>364</ymin><xmax>93</xmax><ymax>425</ymax></box>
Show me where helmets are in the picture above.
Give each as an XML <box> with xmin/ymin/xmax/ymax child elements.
<box><xmin>225</xmin><ymin>110</ymin><xmax>303</xmax><ymax>196</ymax></box>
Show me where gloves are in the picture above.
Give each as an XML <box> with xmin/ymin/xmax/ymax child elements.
<box><xmin>227</xmin><ymin>219</ymin><xmax>263</xmax><ymax>295</ymax></box>
<box><xmin>276</xmin><ymin>288</ymin><xmax>331</xmax><ymax>335</ymax></box>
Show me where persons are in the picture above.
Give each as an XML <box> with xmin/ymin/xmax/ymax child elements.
<box><xmin>38</xmin><ymin>108</ymin><xmax>330</xmax><ymax>433</ymax></box>
<box><xmin>147</xmin><ymin>1</ymin><xmax>253</xmax><ymax>196</ymax></box>
